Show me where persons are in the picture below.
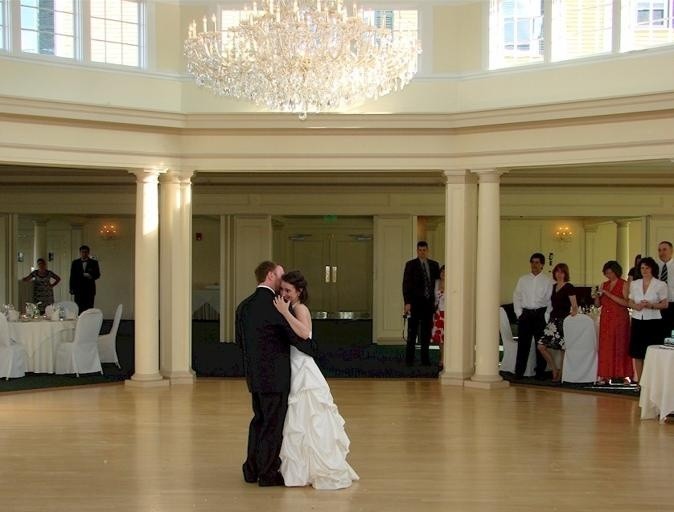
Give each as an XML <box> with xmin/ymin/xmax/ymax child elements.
<box><xmin>272</xmin><ymin>269</ymin><xmax>361</xmax><ymax>488</ymax></box>
<box><xmin>235</xmin><ymin>261</ymin><xmax>318</xmax><ymax>487</ymax></box>
<box><xmin>23</xmin><ymin>258</ymin><xmax>62</xmax><ymax>313</ymax></box>
<box><xmin>629</xmin><ymin>257</ymin><xmax>669</xmax><ymax>393</ymax></box>
<box><xmin>513</xmin><ymin>253</ymin><xmax>553</xmax><ymax>380</ymax></box>
<box><xmin>593</xmin><ymin>260</ymin><xmax>635</xmax><ymax>385</ymax></box>
<box><xmin>656</xmin><ymin>241</ymin><xmax>674</xmax><ymax>337</ymax></box>
<box><xmin>628</xmin><ymin>253</ymin><xmax>642</xmax><ymax>280</ymax></box>
<box><xmin>402</xmin><ymin>239</ymin><xmax>440</xmax><ymax>366</ymax></box>
<box><xmin>538</xmin><ymin>262</ymin><xmax>578</xmax><ymax>382</ymax></box>
<box><xmin>69</xmin><ymin>245</ymin><xmax>101</xmax><ymax>315</ymax></box>
<box><xmin>429</xmin><ymin>265</ymin><xmax>445</xmax><ymax>367</ymax></box>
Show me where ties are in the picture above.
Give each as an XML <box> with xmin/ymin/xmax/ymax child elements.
<box><xmin>421</xmin><ymin>261</ymin><xmax>430</xmax><ymax>286</ymax></box>
<box><xmin>660</xmin><ymin>262</ymin><xmax>669</xmax><ymax>287</ymax></box>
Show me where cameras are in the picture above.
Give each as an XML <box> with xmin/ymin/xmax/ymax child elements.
<box><xmin>403</xmin><ymin>313</ymin><xmax>410</xmax><ymax>319</ymax></box>
<box><xmin>595</xmin><ymin>286</ymin><xmax>602</xmax><ymax>297</ymax></box>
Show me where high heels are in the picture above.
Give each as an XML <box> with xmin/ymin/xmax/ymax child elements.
<box><xmin>552</xmin><ymin>367</ymin><xmax>564</xmax><ymax>382</ymax></box>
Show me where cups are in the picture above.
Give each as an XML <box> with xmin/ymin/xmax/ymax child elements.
<box><xmin>316</xmin><ymin>310</ymin><xmax>360</xmax><ymax>319</ymax></box>
<box><xmin>2</xmin><ymin>304</ymin><xmax>64</xmax><ymax>322</ymax></box>
<box><xmin>569</xmin><ymin>304</ymin><xmax>600</xmax><ymax>316</ymax></box>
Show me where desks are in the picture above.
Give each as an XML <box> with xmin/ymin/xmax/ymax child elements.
<box><xmin>189</xmin><ymin>286</ymin><xmax>222</xmax><ymax>323</ymax></box>
<box><xmin>635</xmin><ymin>342</ymin><xmax>674</xmax><ymax>426</ymax></box>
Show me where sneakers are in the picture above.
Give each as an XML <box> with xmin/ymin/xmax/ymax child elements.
<box><xmin>596</xmin><ymin>374</ymin><xmax>645</xmax><ymax>391</ymax></box>
<box><xmin>510</xmin><ymin>369</ymin><xmax>548</xmax><ymax>380</ymax></box>
<box><xmin>402</xmin><ymin>356</ymin><xmax>446</xmax><ymax>369</ymax></box>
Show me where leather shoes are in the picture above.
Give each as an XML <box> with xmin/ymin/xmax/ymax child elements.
<box><xmin>242</xmin><ymin>469</ymin><xmax>288</xmax><ymax>487</ymax></box>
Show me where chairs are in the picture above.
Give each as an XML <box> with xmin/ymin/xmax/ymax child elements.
<box><xmin>560</xmin><ymin>311</ymin><xmax>609</xmax><ymax>384</ymax></box>
<box><xmin>0</xmin><ymin>300</ymin><xmax>125</xmax><ymax>383</ymax></box>
<box><xmin>498</xmin><ymin>303</ymin><xmax>544</xmax><ymax>376</ymax></box>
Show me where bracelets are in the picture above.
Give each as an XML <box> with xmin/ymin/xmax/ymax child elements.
<box><xmin>651</xmin><ymin>304</ymin><xmax>654</xmax><ymax>309</ymax></box>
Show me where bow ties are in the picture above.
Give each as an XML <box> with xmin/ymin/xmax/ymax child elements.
<box><xmin>82</xmin><ymin>259</ymin><xmax>88</xmax><ymax>262</ymax></box>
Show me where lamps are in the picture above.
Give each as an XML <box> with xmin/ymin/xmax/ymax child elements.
<box><xmin>98</xmin><ymin>222</ymin><xmax>116</xmax><ymax>238</ymax></box>
<box><xmin>180</xmin><ymin>0</ymin><xmax>423</xmax><ymax>123</ymax></box>
<box><xmin>553</xmin><ymin>226</ymin><xmax>574</xmax><ymax>243</ymax></box>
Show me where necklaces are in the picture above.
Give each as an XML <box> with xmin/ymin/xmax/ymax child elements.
<box><xmin>291</xmin><ymin>300</ymin><xmax>301</xmax><ymax>310</ymax></box>
